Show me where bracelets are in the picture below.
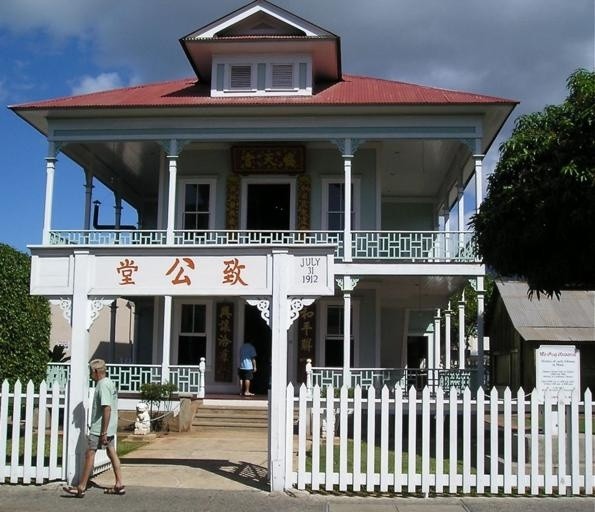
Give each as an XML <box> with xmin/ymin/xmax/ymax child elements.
<box><xmin>101</xmin><ymin>431</ymin><xmax>107</xmax><ymax>436</ymax></box>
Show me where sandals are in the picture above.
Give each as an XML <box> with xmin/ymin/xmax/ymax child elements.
<box><xmin>104</xmin><ymin>484</ymin><xmax>125</xmax><ymax>495</ymax></box>
<box><xmin>244</xmin><ymin>392</ymin><xmax>255</xmax><ymax>397</ymax></box>
<box><xmin>63</xmin><ymin>485</ymin><xmax>86</xmax><ymax>497</ymax></box>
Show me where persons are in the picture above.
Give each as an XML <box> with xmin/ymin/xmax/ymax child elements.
<box><xmin>237</xmin><ymin>335</ymin><xmax>258</xmax><ymax>397</ymax></box>
<box><xmin>63</xmin><ymin>356</ymin><xmax>127</xmax><ymax>496</ymax></box>
